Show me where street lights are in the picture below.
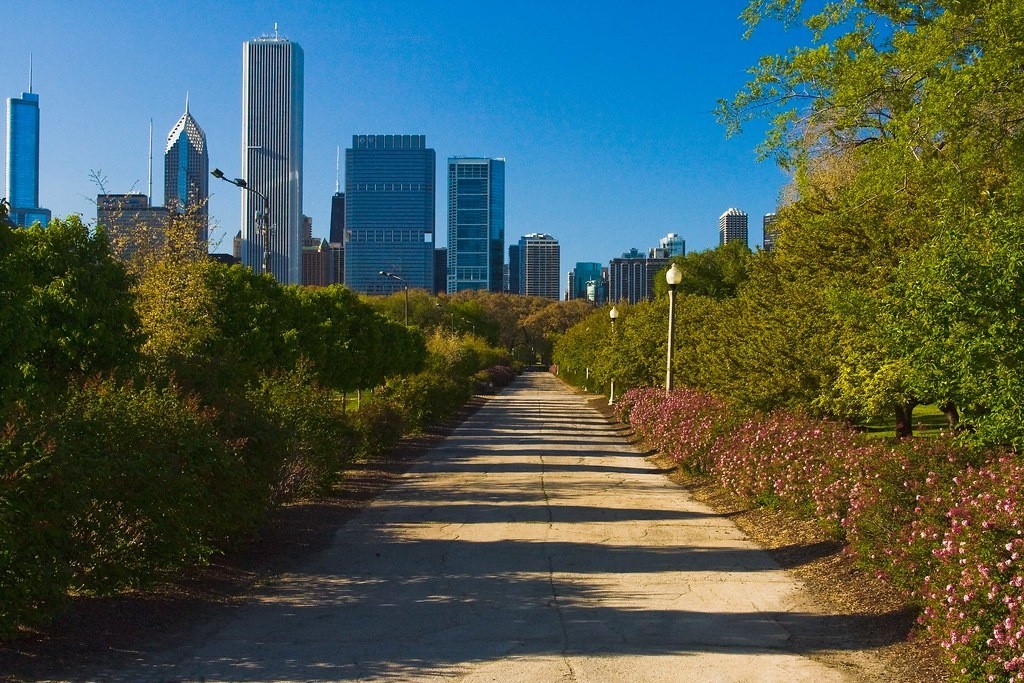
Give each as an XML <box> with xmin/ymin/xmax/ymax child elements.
<box><xmin>607</xmin><ymin>305</ymin><xmax>620</xmax><ymax>406</ymax></box>
<box><xmin>461</xmin><ymin>316</ymin><xmax>477</xmax><ymax>339</ymax></box>
<box><xmin>379</xmin><ymin>270</ymin><xmax>409</xmax><ymax>327</ymax></box>
<box><xmin>665</xmin><ymin>261</ymin><xmax>683</xmax><ymax>399</ymax></box>
<box><xmin>436</xmin><ymin>303</ymin><xmax>454</xmax><ymax>334</ymax></box>
<box><xmin>210</xmin><ymin>168</ymin><xmax>276</xmax><ymax>273</ymax></box>
